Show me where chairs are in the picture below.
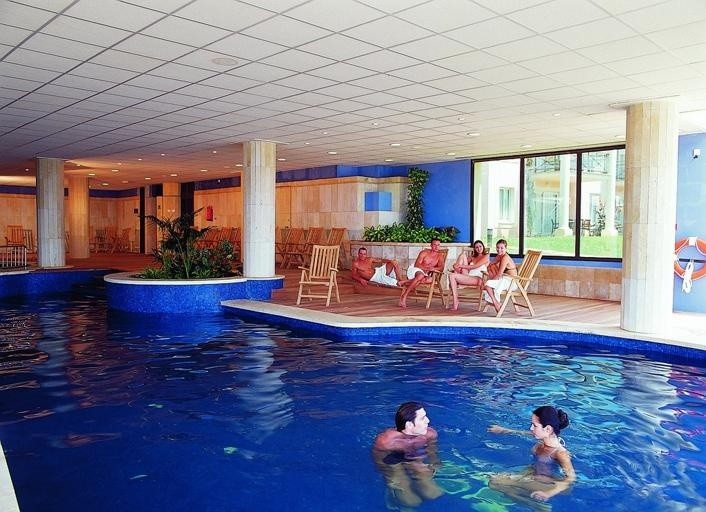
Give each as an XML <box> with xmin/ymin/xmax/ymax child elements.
<box><xmin>8</xmin><ymin>224</ymin><xmax>134</xmax><ymax>256</ymax></box>
<box><xmin>296</xmin><ymin>244</ymin><xmax>344</xmax><ymax>306</ymax></box>
<box><xmin>184</xmin><ymin>226</ymin><xmax>349</xmax><ymax>270</ymax></box>
<box><xmin>351</xmin><ymin>246</ymin><xmax>543</xmax><ymax>318</ymax></box>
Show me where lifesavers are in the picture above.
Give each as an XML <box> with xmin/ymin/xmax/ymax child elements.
<box><xmin>674</xmin><ymin>236</ymin><xmax>706</xmax><ymax>281</ymax></box>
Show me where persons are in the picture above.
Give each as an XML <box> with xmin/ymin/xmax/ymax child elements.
<box><xmin>448</xmin><ymin>240</ymin><xmax>490</xmax><ymax>310</ymax></box>
<box><xmin>486</xmin><ymin>405</ymin><xmax>576</xmax><ymax>512</ymax></box>
<box><xmin>398</xmin><ymin>238</ymin><xmax>445</xmax><ymax>308</ymax></box>
<box><xmin>371</xmin><ymin>401</ymin><xmax>445</xmax><ymax>508</ymax></box>
<box><xmin>351</xmin><ymin>247</ymin><xmax>406</xmax><ymax>288</ymax></box>
<box><xmin>481</xmin><ymin>239</ymin><xmax>519</xmax><ymax>314</ymax></box>
